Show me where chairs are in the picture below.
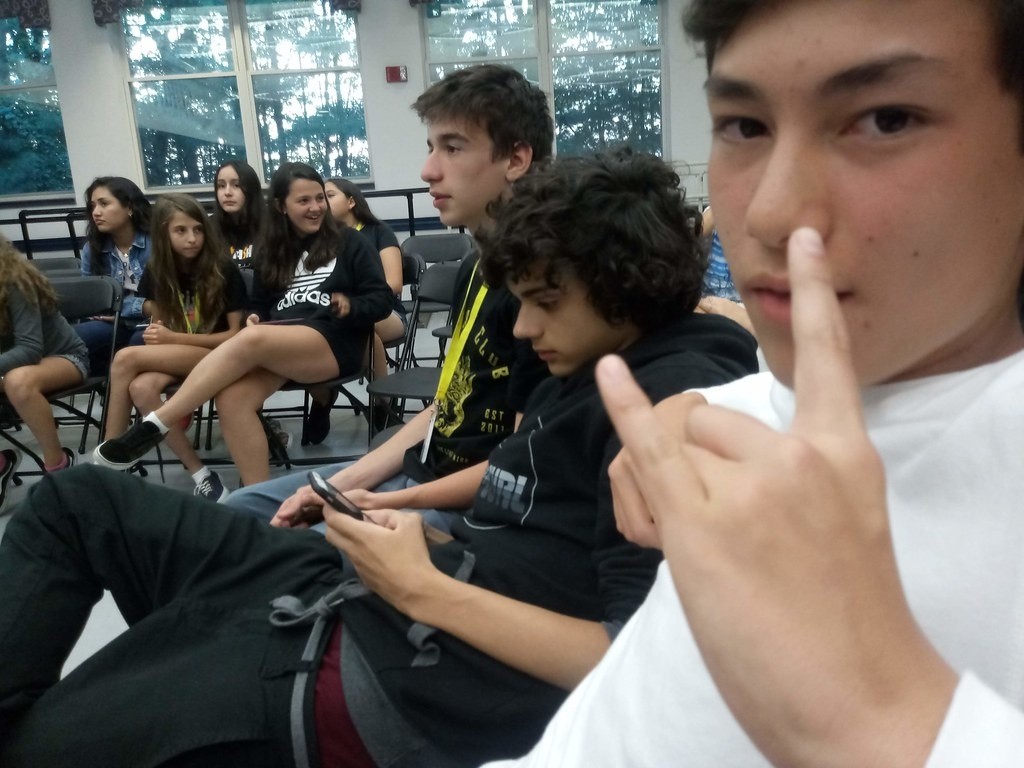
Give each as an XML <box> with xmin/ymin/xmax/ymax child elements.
<box><xmin>1</xmin><ymin>235</ymin><xmax>474</xmax><ymax>485</ymax></box>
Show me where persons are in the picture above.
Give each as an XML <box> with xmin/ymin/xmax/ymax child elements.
<box><xmin>478</xmin><ymin>0</ymin><xmax>1024</xmax><ymax>768</ymax></box>
<box><xmin>0</xmin><ymin>161</ymin><xmax>408</xmax><ymax>513</ymax></box>
<box><xmin>0</xmin><ymin>144</ymin><xmax>760</xmax><ymax>768</ymax></box>
<box><xmin>218</xmin><ymin>66</ymin><xmax>555</xmax><ymax>577</ymax></box>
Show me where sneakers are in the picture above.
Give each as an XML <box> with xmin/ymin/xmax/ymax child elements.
<box><xmin>42</xmin><ymin>447</ymin><xmax>77</xmax><ymax>478</ymax></box>
<box><xmin>0</xmin><ymin>449</ymin><xmax>22</xmax><ymax>516</ymax></box>
<box><xmin>193</xmin><ymin>468</ymin><xmax>229</xmax><ymax>505</ymax></box>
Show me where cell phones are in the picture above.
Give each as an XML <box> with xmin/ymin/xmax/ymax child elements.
<box><xmin>135</xmin><ymin>316</ymin><xmax>153</xmax><ymax>329</ymax></box>
<box><xmin>307</xmin><ymin>470</ymin><xmax>366</xmax><ymax>520</ymax></box>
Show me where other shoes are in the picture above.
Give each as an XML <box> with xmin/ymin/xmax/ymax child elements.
<box><xmin>268</xmin><ymin>416</ymin><xmax>293</xmax><ymax>452</ymax></box>
<box><xmin>374</xmin><ymin>402</ymin><xmax>405</xmax><ymax>433</ymax></box>
<box><xmin>94</xmin><ymin>422</ymin><xmax>169</xmax><ymax>471</ymax></box>
<box><xmin>308</xmin><ymin>385</ymin><xmax>339</xmax><ymax>446</ymax></box>
<box><xmin>166</xmin><ymin>390</ymin><xmax>193</xmax><ymax>433</ymax></box>
